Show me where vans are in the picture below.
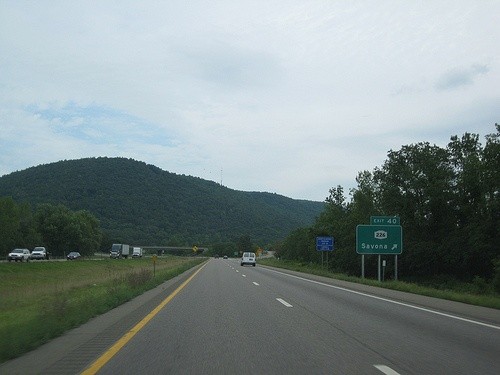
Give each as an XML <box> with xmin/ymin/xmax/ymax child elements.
<box><xmin>241</xmin><ymin>253</ymin><xmax>256</xmax><ymax>267</ymax></box>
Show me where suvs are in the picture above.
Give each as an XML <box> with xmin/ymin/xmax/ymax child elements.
<box><xmin>8</xmin><ymin>249</ymin><xmax>31</xmax><ymax>262</ymax></box>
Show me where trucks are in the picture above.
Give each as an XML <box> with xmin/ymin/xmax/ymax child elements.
<box><xmin>31</xmin><ymin>246</ymin><xmax>50</xmax><ymax>260</ymax></box>
<box><xmin>131</xmin><ymin>247</ymin><xmax>143</xmax><ymax>259</ymax></box>
<box><xmin>110</xmin><ymin>243</ymin><xmax>129</xmax><ymax>259</ymax></box>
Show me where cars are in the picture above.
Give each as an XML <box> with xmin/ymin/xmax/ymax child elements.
<box><xmin>66</xmin><ymin>252</ymin><xmax>81</xmax><ymax>261</ymax></box>
<box><xmin>214</xmin><ymin>254</ymin><xmax>219</xmax><ymax>259</ymax></box>
<box><xmin>223</xmin><ymin>256</ymin><xmax>228</xmax><ymax>259</ymax></box>
